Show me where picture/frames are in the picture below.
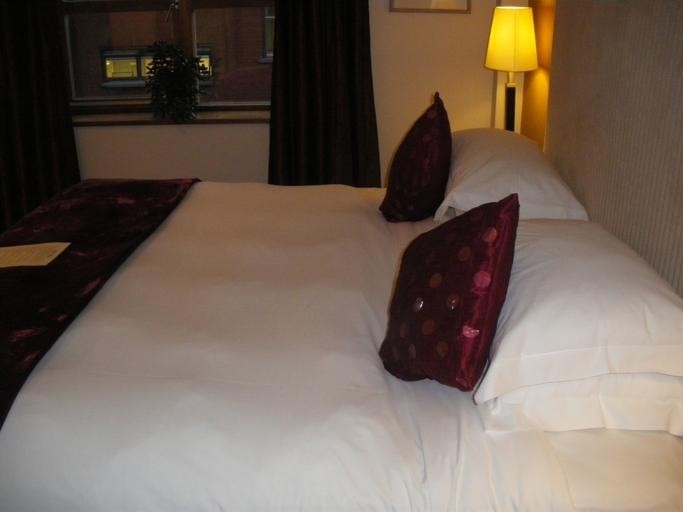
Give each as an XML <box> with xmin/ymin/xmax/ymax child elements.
<box><xmin>389</xmin><ymin>1</ymin><xmax>472</xmax><ymax>14</ymax></box>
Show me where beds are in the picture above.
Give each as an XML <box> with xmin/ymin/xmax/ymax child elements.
<box><xmin>1</xmin><ymin>176</ymin><xmax>682</xmax><ymax>511</ymax></box>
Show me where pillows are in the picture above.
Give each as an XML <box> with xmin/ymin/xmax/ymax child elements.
<box><xmin>380</xmin><ymin>192</ymin><xmax>517</xmax><ymax>386</ymax></box>
<box><xmin>467</xmin><ymin>217</ymin><xmax>682</xmax><ymax>439</ymax></box>
<box><xmin>378</xmin><ymin>93</ymin><xmax>451</xmax><ymax>223</ymax></box>
<box><xmin>431</xmin><ymin>125</ymin><xmax>591</xmax><ymax>221</ymax></box>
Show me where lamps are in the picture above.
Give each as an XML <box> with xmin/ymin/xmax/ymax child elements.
<box><xmin>485</xmin><ymin>6</ymin><xmax>540</xmax><ymax>131</ymax></box>
